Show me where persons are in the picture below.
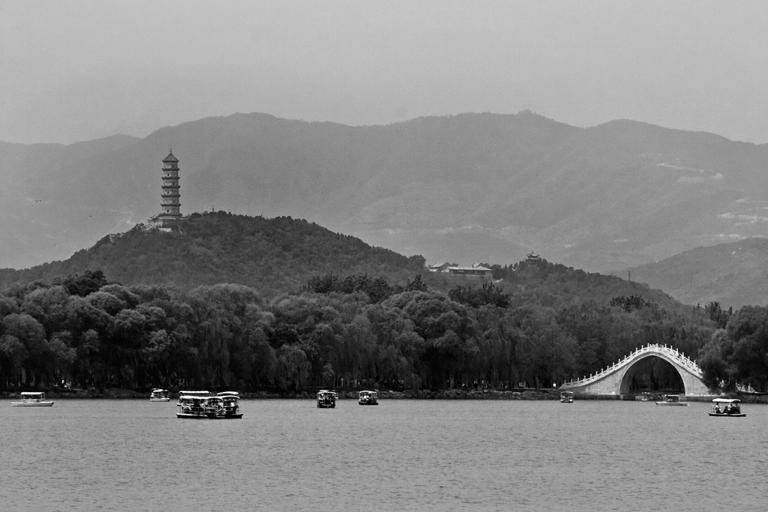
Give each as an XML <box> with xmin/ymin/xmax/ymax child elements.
<box><xmin>713</xmin><ymin>402</ymin><xmax>741</xmax><ymax>415</ymax></box>
<box><xmin>179</xmin><ymin>396</ymin><xmax>239</xmax><ymax>416</ymax></box>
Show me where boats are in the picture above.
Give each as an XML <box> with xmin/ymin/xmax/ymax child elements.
<box><xmin>318</xmin><ymin>389</ymin><xmax>338</xmax><ymax>409</ymax></box>
<box><xmin>654</xmin><ymin>395</ymin><xmax>689</xmax><ymax>407</ymax></box>
<box><xmin>703</xmin><ymin>398</ymin><xmax>747</xmax><ymax>417</ymax></box>
<box><xmin>149</xmin><ymin>387</ymin><xmax>244</xmax><ymax>420</ymax></box>
<box><xmin>358</xmin><ymin>390</ymin><xmax>379</xmax><ymax>406</ymax></box>
<box><xmin>560</xmin><ymin>389</ymin><xmax>573</xmax><ymax>404</ymax></box>
<box><xmin>10</xmin><ymin>390</ymin><xmax>54</xmax><ymax>407</ymax></box>
